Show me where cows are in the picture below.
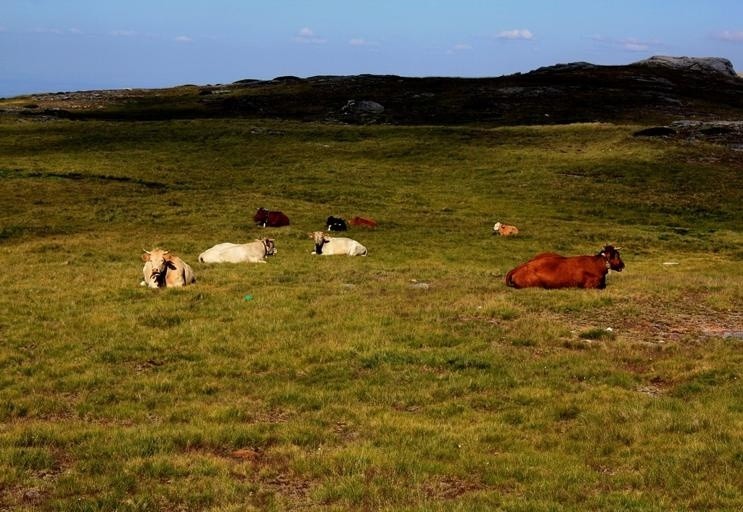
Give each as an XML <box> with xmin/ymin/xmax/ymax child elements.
<box><xmin>137</xmin><ymin>247</ymin><xmax>195</xmax><ymax>289</ymax></box>
<box><xmin>347</xmin><ymin>215</ymin><xmax>379</xmax><ymax>229</ymax></box>
<box><xmin>253</xmin><ymin>207</ymin><xmax>290</xmax><ymax>227</ymax></box>
<box><xmin>506</xmin><ymin>241</ymin><xmax>625</xmax><ymax>290</ymax></box>
<box><xmin>307</xmin><ymin>231</ymin><xmax>367</xmax><ymax>257</ymax></box>
<box><xmin>492</xmin><ymin>222</ymin><xmax>519</xmax><ymax>236</ymax></box>
<box><xmin>324</xmin><ymin>216</ymin><xmax>347</xmax><ymax>231</ymax></box>
<box><xmin>198</xmin><ymin>236</ymin><xmax>278</xmax><ymax>264</ymax></box>
